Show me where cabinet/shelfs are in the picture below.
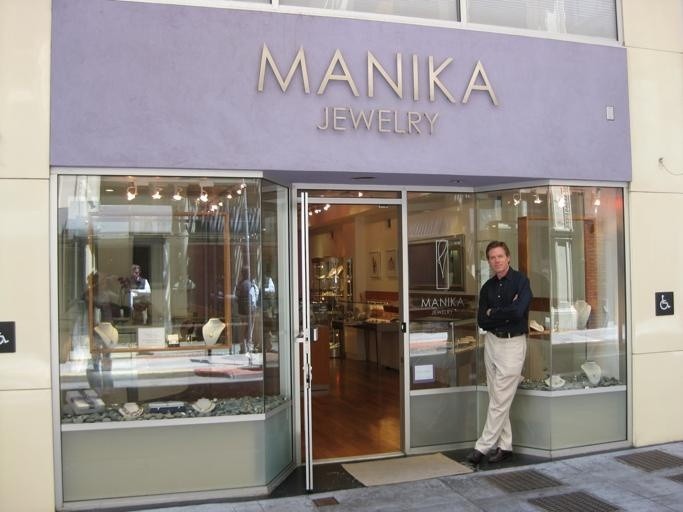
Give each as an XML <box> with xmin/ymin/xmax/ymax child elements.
<box><xmin>58</xmin><ymin>349</ymin><xmax>291</xmax><ymax>501</ymax></box>
<box><xmin>311</xmin><ymin>256</ymin><xmax>353</xmax><ymax>358</ymax></box>
<box><xmin>478</xmin><ymin>328</ymin><xmax>627</xmax><ymax>451</ymax></box>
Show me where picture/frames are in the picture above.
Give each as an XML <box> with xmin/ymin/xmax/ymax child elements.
<box><xmin>366</xmin><ymin>249</ymin><xmax>381</xmax><ymax>280</ymax></box>
<box><xmin>386</xmin><ymin>250</ymin><xmax>398</xmax><ymax>279</ymax></box>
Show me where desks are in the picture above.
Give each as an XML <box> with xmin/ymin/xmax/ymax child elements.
<box><xmin>343</xmin><ymin>318</ymin><xmax>477</xmax><ymax>387</ymax></box>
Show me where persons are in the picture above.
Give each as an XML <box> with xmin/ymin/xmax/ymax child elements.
<box><xmin>236</xmin><ymin>265</ymin><xmax>262</xmax><ymax>351</ymax></box>
<box><xmin>125</xmin><ymin>265</ymin><xmax>151</xmax><ymax>324</ymax></box>
<box><xmin>465</xmin><ymin>241</ymin><xmax>531</xmax><ymax>462</ymax></box>
<box><xmin>252</xmin><ymin>266</ymin><xmax>275</xmax><ymax>352</ymax></box>
<box><xmin>77</xmin><ymin>274</ymin><xmax>126</xmax><ymax>392</ymax></box>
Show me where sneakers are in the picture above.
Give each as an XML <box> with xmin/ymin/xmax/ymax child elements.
<box><xmin>464</xmin><ymin>448</ymin><xmax>484</xmax><ymax>465</ymax></box>
<box><xmin>488</xmin><ymin>448</ymin><xmax>512</xmax><ymax>463</ymax></box>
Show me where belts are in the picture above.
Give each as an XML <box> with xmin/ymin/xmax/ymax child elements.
<box><xmin>491</xmin><ymin>329</ymin><xmax>525</xmax><ymax>338</ymax></box>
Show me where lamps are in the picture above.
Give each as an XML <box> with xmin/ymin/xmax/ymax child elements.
<box><xmin>124</xmin><ymin>179</ymin><xmax>209</xmax><ymax>205</ymax></box>
<box><xmin>508</xmin><ymin>187</ymin><xmax>601</xmax><ymax>208</ymax></box>
<box><xmin>516</xmin><ymin>192</ymin><xmax>530</xmax><ymax>219</ymax></box>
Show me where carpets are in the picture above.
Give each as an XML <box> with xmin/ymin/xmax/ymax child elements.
<box><xmin>340</xmin><ymin>450</ymin><xmax>475</xmax><ymax>488</ymax></box>
